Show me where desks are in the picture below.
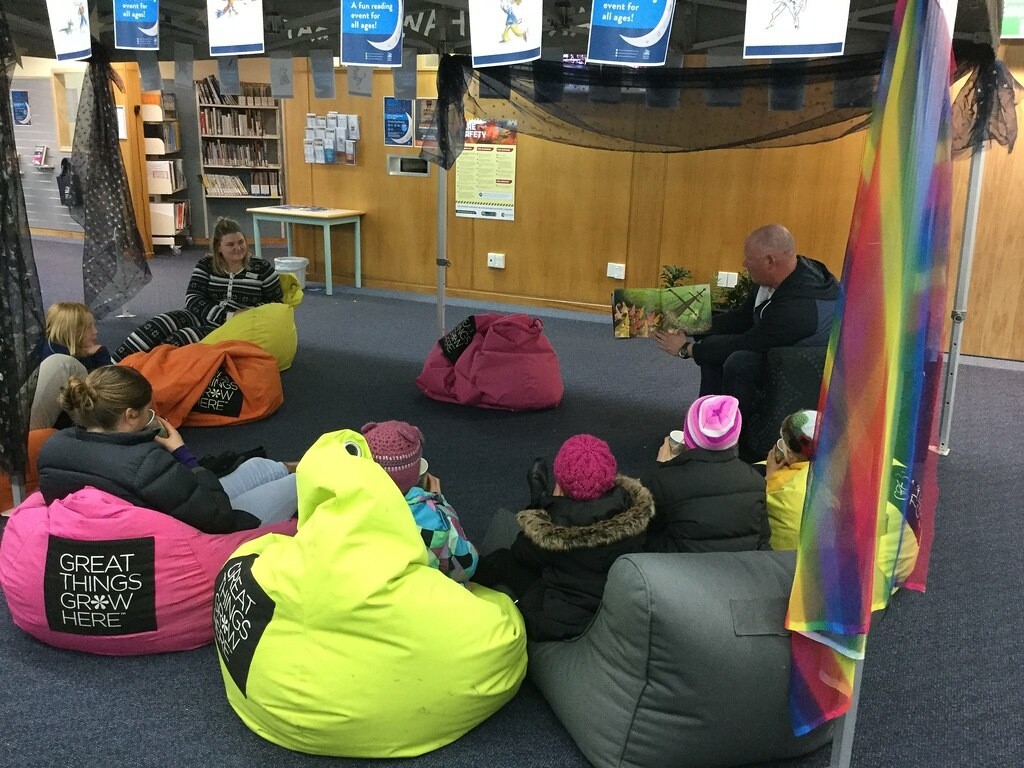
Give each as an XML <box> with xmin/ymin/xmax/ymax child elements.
<box><xmin>245</xmin><ymin>204</ymin><xmax>365</xmax><ymax>296</ymax></box>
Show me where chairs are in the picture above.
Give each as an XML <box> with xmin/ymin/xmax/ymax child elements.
<box><xmin>478</xmin><ymin>508</ymin><xmax>833</xmax><ymax>768</ymax></box>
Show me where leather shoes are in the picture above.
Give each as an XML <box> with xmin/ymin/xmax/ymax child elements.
<box><xmin>527</xmin><ymin>458</ymin><xmax>553</xmax><ymax>510</ymax></box>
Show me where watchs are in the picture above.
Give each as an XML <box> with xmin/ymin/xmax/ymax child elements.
<box><xmin>678</xmin><ymin>341</ymin><xmax>691</xmax><ymax>359</ymax></box>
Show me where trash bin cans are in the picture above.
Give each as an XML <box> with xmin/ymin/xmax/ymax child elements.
<box><xmin>273</xmin><ymin>257</ymin><xmax>309</xmax><ymax>290</ymax></box>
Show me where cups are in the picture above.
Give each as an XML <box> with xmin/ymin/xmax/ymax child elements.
<box><xmin>143</xmin><ymin>408</ymin><xmax>168</xmax><ymax>439</ymax></box>
<box><xmin>775</xmin><ymin>439</ymin><xmax>786</xmax><ymax>464</ymax></box>
<box><xmin>670</xmin><ymin>430</ymin><xmax>685</xmax><ymax>454</ymax></box>
<box><xmin>419</xmin><ymin>458</ymin><xmax>429</xmax><ymax>492</ymax></box>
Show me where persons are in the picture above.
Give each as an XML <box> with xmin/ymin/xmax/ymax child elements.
<box><xmin>111</xmin><ymin>216</ymin><xmax>284</xmax><ymax>365</ymax></box>
<box><xmin>764</xmin><ymin>409</ymin><xmax>920</xmax><ymax>614</ymax></box>
<box><xmin>641</xmin><ymin>394</ymin><xmax>771</xmax><ymax>553</ymax></box>
<box><xmin>651</xmin><ymin>224</ymin><xmax>840</xmax><ymax>465</ymax></box>
<box><xmin>16</xmin><ymin>302</ymin><xmax>112</xmax><ymax>430</ymax></box>
<box><xmin>36</xmin><ymin>365</ymin><xmax>297</xmax><ymax>535</ymax></box>
<box><xmin>480</xmin><ymin>394</ymin><xmax>655</xmax><ymax>641</ymax></box>
<box><xmin>360</xmin><ymin>420</ymin><xmax>479</xmax><ymax>592</ymax></box>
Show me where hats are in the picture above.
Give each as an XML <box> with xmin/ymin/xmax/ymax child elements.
<box><xmin>683</xmin><ymin>395</ymin><xmax>742</xmax><ymax>452</ymax></box>
<box><xmin>554</xmin><ymin>434</ymin><xmax>616</xmax><ymax>499</ymax></box>
<box><xmin>780</xmin><ymin>409</ymin><xmax>818</xmax><ymax>462</ymax></box>
<box><xmin>360</xmin><ymin>421</ymin><xmax>425</xmax><ymax>494</ymax></box>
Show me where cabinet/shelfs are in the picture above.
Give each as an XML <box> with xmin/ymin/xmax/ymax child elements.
<box><xmin>194</xmin><ymin>84</ymin><xmax>286</xmax><ymax>240</ymax></box>
<box><xmin>143</xmin><ymin>118</ymin><xmax>194</xmax><ymax>256</ymax></box>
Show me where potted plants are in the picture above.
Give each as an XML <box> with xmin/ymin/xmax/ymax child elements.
<box><xmin>658</xmin><ymin>265</ymin><xmax>754</xmax><ymax>314</ymax></box>
